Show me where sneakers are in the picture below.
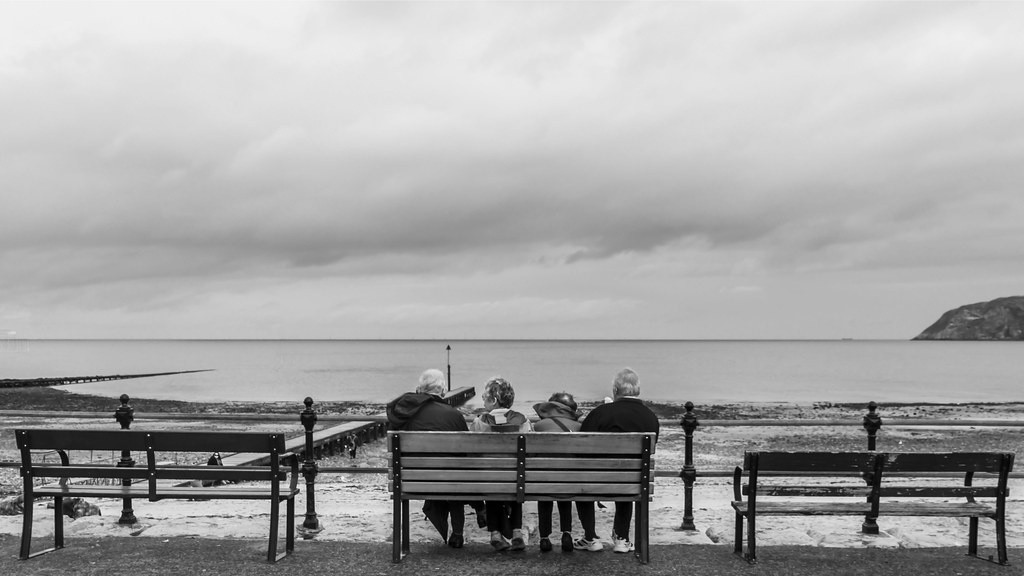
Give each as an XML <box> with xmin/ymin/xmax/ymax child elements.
<box><xmin>511</xmin><ymin>529</ymin><xmax>526</xmax><ymax>553</ymax></box>
<box><xmin>613</xmin><ymin>535</ymin><xmax>636</xmax><ymax>553</ymax></box>
<box><xmin>490</xmin><ymin>530</ymin><xmax>511</xmax><ymax>551</ymax></box>
<box><xmin>573</xmin><ymin>536</ymin><xmax>603</xmax><ymax>552</ymax></box>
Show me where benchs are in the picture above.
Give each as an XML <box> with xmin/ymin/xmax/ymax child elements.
<box><xmin>731</xmin><ymin>449</ymin><xmax>1016</xmax><ymax>564</ymax></box>
<box><xmin>386</xmin><ymin>429</ymin><xmax>657</xmax><ymax>564</ymax></box>
<box><xmin>13</xmin><ymin>428</ymin><xmax>300</xmax><ymax>562</ymax></box>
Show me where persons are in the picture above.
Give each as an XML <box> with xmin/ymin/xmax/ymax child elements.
<box><xmin>533</xmin><ymin>392</ymin><xmax>581</xmax><ymax>554</ymax></box>
<box><xmin>572</xmin><ymin>368</ymin><xmax>659</xmax><ymax>554</ymax></box>
<box><xmin>469</xmin><ymin>378</ymin><xmax>532</xmax><ymax>552</ymax></box>
<box><xmin>386</xmin><ymin>368</ymin><xmax>469</xmax><ymax>549</ymax></box>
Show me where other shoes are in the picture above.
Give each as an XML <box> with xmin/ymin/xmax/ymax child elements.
<box><xmin>476</xmin><ymin>509</ymin><xmax>488</xmax><ymax>529</ymax></box>
<box><xmin>448</xmin><ymin>532</ymin><xmax>464</xmax><ymax>548</ymax></box>
<box><xmin>540</xmin><ymin>538</ymin><xmax>553</xmax><ymax>552</ymax></box>
<box><xmin>561</xmin><ymin>532</ymin><xmax>574</xmax><ymax>553</ymax></box>
